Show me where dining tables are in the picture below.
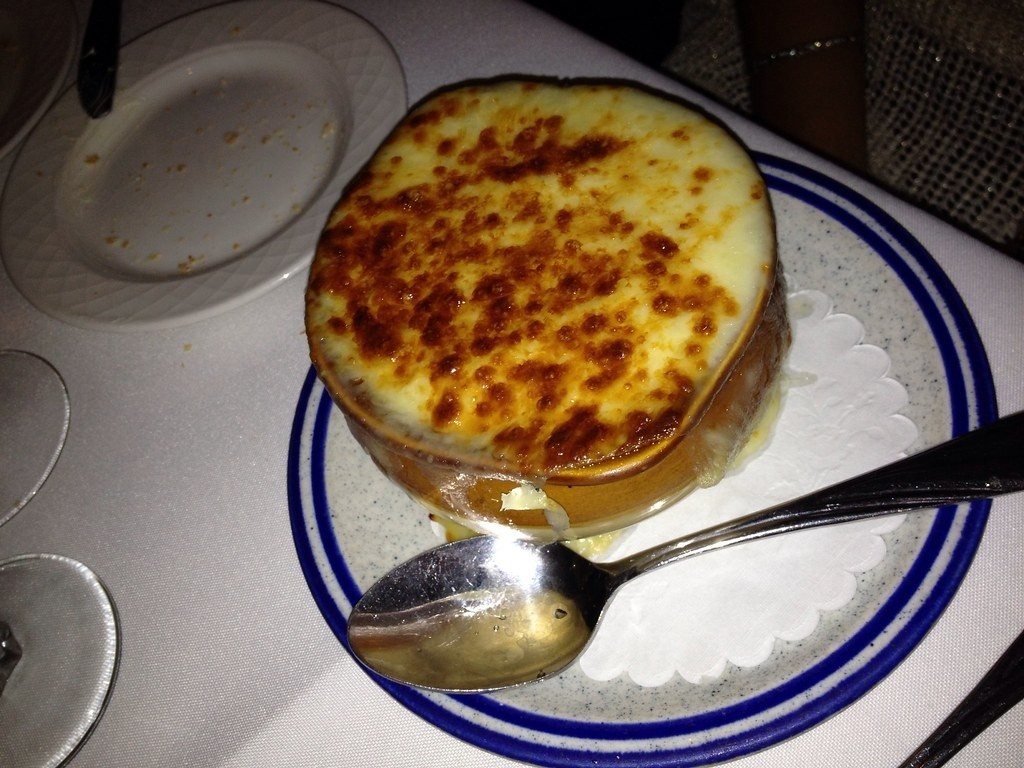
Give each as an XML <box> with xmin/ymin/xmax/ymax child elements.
<box><xmin>1</xmin><ymin>1</ymin><xmax>1024</xmax><ymax>768</ymax></box>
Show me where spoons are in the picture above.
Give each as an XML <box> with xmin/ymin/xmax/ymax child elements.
<box><xmin>346</xmin><ymin>411</ymin><xmax>1024</xmax><ymax>694</ymax></box>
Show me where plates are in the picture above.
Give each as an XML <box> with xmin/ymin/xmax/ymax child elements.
<box><xmin>288</xmin><ymin>151</ymin><xmax>996</xmax><ymax>768</ymax></box>
<box><xmin>1</xmin><ymin>0</ymin><xmax>408</xmax><ymax>328</ymax></box>
<box><xmin>0</xmin><ymin>0</ymin><xmax>78</xmax><ymax>160</ymax></box>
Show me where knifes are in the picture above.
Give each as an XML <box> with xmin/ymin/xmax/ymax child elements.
<box><xmin>77</xmin><ymin>0</ymin><xmax>123</xmax><ymax>118</ymax></box>
<box><xmin>894</xmin><ymin>633</ymin><xmax>1022</xmax><ymax>767</ymax></box>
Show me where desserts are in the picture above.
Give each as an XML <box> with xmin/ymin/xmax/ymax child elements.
<box><xmin>306</xmin><ymin>73</ymin><xmax>792</xmax><ymax>541</ymax></box>
